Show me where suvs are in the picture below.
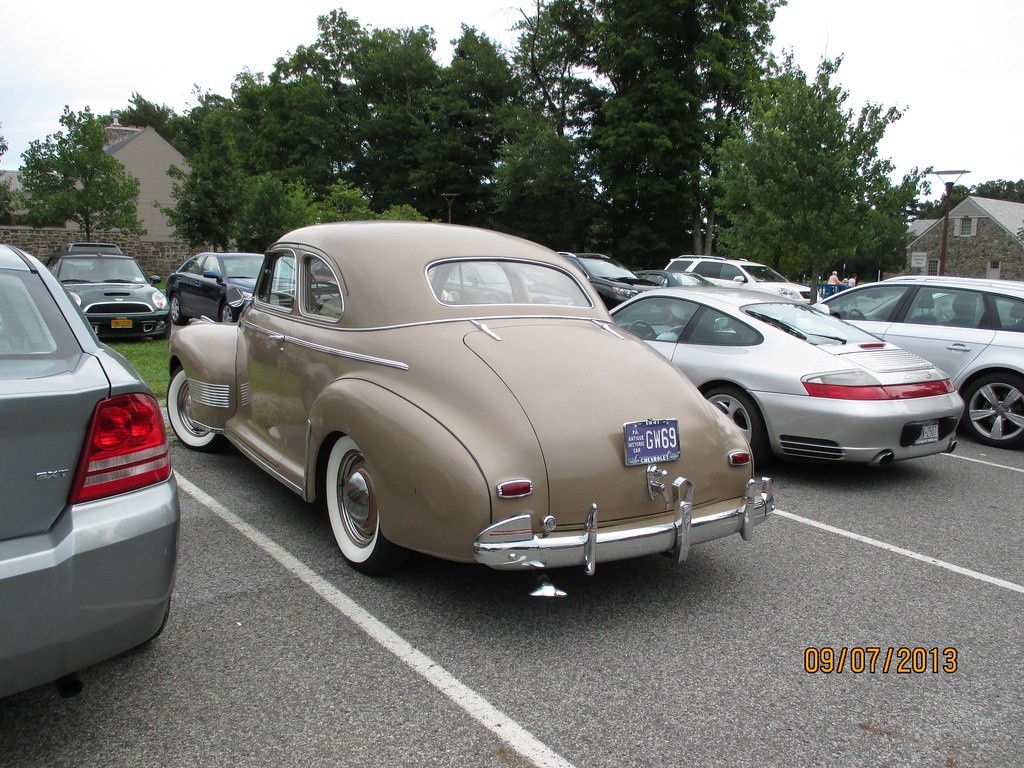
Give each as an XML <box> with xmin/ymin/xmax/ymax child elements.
<box><xmin>662</xmin><ymin>255</ymin><xmax>821</xmax><ymax>305</ymax></box>
<box><xmin>557</xmin><ymin>249</ymin><xmax>672</xmax><ymax>312</ymax></box>
<box><xmin>809</xmin><ymin>275</ymin><xmax>1024</xmax><ymax>448</ymax></box>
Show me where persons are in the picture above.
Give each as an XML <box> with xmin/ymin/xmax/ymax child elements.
<box><xmin>744</xmin><ymin>268</ymin><xmax>751</xmax><ymax>275</ymax></box>
<box><xmin>827</xmin><ymin>271</ymin><xmax>859</xmax><ymax>292</ymax></box>
<box><xmin>656</xmin><ymin>303</ymin><xmax>691</xmax><ymax>341</ymax></box>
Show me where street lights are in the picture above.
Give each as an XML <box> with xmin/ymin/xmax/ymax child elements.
<box><xmin>931</xmin><ymin>169</ymin><xmax>972</xmax><ymax>276</ymax></box>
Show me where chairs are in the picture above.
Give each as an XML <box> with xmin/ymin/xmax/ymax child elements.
<box><xmin>947</xmin><ymin>294</ymin><xmax>973</xmax><ymax>325</ymax></box>
<box><xmin>910</xmin><ymin>292</ymin><xmax>936</xmax><ymax>322</ymax></box>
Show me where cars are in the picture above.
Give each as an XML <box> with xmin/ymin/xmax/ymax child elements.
<box><xmin>43</xmin><ymin>242</ymin><xmax>125</xmax><ymax>270</ymax></box>
<box><xmin>309</xmin><ymin>256</ymin><xmax>576</xmax><ymax>310</ymax></box>
<box><xmin>49</xmin><ymin>254</ymin><xmax>172</xmax><ymax>341</ymax></box>
<box><xmin>606</xmin><ymin>286</ymin><xmax>967</xmax><ymax>474</ymax></box>
<box><xmin>630</xmin><ymin>267</ymin><xmax>713</xmax><ymax>288</ymax></box>
<box><xmin>163</xmin><ymin>254</ymin><xmax>324</xmax><ymax>327</ymax></box>
<box><xmin>0</xmin><ymin>243</ymin><xmax>179</xmax><ymax>701</ymax></box>
<box><xmin>164</xmin><ymin>220</ymin><xmax>777</xmax><ymax>580</ymax></box>
